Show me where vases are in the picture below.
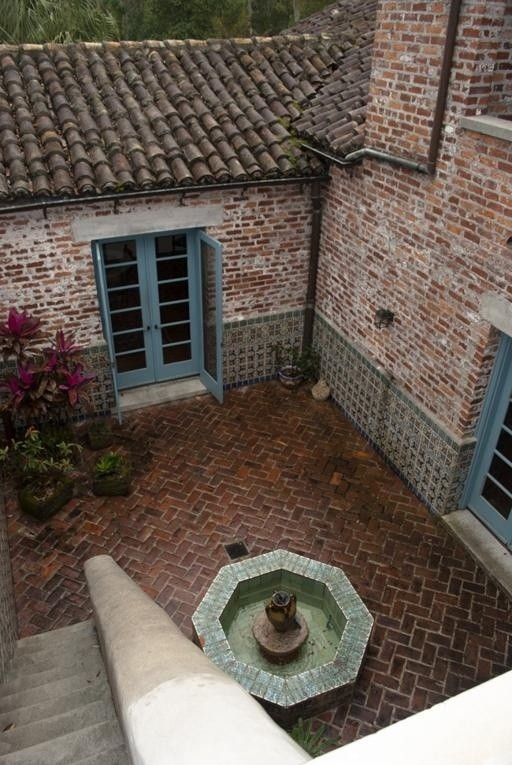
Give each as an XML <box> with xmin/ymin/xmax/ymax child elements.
<box><xmin>37</xmin><ymin>427</ymin><xmax>80</xmax><ymax>466</ymax></box>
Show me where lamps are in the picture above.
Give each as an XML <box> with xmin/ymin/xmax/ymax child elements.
<box><xmin>373</xmin><ymin>309</ymin><xmax>394</xmax><ymax>329</ymax></box>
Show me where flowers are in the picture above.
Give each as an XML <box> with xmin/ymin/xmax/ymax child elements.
<box><xmin>0</xmin><ymin>308</ymin><xmax>96</xmax><ymax>427</ymax></box>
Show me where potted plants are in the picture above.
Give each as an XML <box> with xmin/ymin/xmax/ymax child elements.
<box><xmin>273</xmin><ymin>341</ymin><xmax>321</xmax><ymax>389</ymax></box>
<box><xmin>92</xmin><ymin>451</ymin><xmax>132</xmax><ymax>496</ymax></box>
<box><xmin>87</xmin><ymin>419</ymin><xmax>113</xmax><ymax>450</ymax></box>
<box><xmin>0</xmin><ymin>427</ymin><xmax>75</xmax><ymax>519</ymax></box>
